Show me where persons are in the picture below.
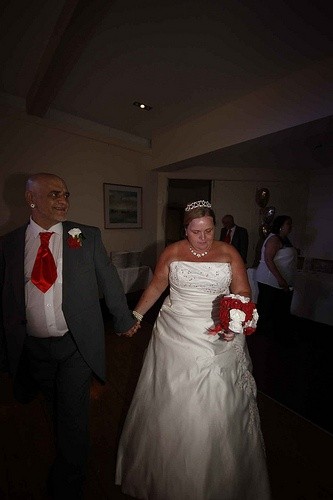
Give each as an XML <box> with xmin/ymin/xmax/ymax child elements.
<box><xmin>254</xmin><ymin>215</ymin><xmax>301</xmax><ymax>335</ymax></box>
<box><xmin>116</xmin><ymin>201</ymin><xmax>270</xmax><ymax>500</ymax></box>
<box><xmin>0</xmin><ymin>172</ymin><xmax>141</xmax><ymax>500</ymax></box>
<box><xmin>219</xmin><ymin>215</ymin><xmax>249</xmax><ymax>266</ymax></box>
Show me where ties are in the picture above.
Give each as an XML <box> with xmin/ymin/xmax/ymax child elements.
<box><xmin>224</xmin><ymin>229</ymin><xmax>232</xmax><ymax>244</ymax></box>
<box><xmin>30</xmin><ymin>231</ymin><xmax>57</xmax><ymax>293</ymax></box>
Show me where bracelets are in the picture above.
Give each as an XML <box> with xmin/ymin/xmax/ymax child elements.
<box><xmin>132</xmin><ymin>310</ymin><xmax>144</xmax><ymax>322</ymax></box>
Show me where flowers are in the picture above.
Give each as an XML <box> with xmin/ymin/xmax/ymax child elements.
<box><xmin>218</xmin><ymin>294</ymin><xmax>259</xmax><ymax>335</ymax></box>
<box><xmin>66</xmin><ymin>228</ymin><xmax>86</xmax><ymax>249</ymax></box>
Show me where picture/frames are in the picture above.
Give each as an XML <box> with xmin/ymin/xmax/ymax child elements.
<box><xmin>103</xmin><ymin>183</ymin><xmax>142</xmax><ymax>229</ymax></box>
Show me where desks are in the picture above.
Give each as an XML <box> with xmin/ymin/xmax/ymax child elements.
<box><xmin>246</xmin><ymin>268</ymin><xmax>333</xmax><ymax>326</ymax></box>
<box><xmin>98</xmin><ymin>258</ymin><xmax>156</xmax><ymax>298</ymax></box>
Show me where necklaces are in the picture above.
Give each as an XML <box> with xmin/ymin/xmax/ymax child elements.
<box><xmin>189</xmin><ymin>244</ymin><xmax>211</xmax><ymax>258</ymax></box>
